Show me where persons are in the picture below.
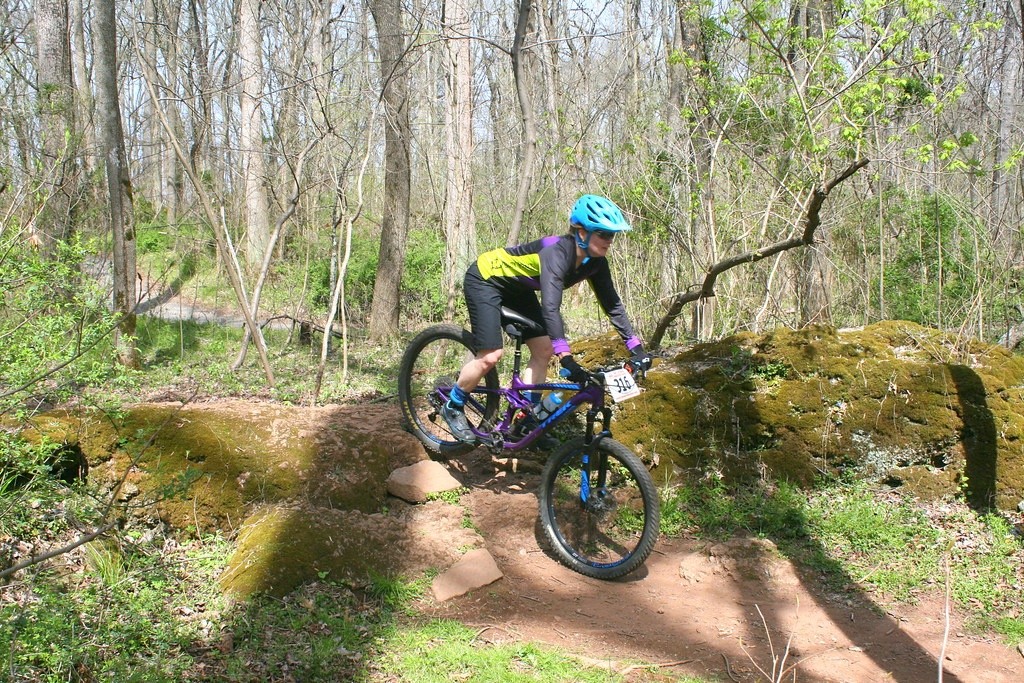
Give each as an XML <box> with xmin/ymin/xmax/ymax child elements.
<box><xmin>439</xmin><ymin>193</ymin><xmax>652</xmax><ymax>446</ymax></box>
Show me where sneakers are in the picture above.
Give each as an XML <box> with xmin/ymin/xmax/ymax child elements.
<box><xmin>506</xmin><ymin>414</ymin><xmax>561</xmax><ymax>449</ymax></box>
<box><xmin>439</xmin><ymin>400</ymin><xmax>478</xmax><ymax>443</ymax></box>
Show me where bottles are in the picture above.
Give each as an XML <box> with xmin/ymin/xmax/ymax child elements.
<box><xmin>534</xmin><ymin>391</ymin><xmax>564</xmax><ymax>420</ymax></box>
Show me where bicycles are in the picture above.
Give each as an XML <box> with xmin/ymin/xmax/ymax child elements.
<box><xmin>398</xmin><ymin>304</ymin><xmax>662</xmax><ymax>581</ymax></box>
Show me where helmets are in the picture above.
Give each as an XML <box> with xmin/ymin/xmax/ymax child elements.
<box><xmin>569</xmin><ymin>194</ymin><xmax>632</xmax><ymax>237</ymax></box>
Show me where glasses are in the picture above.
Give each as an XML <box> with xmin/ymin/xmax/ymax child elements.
<box><xmin>593</xmin><ymin>230</ymin><xmax>616</xmax><ymax>240</ymax></box>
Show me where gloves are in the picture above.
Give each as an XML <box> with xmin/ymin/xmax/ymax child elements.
<box><xmin>629</xmin><ymin>345</ymin><xmax>653</xmax><ymax>373</ymax></box>
<box><xmin>559</xmin><ymin>355</ymin><xmax>590</xmax><ymax>382</ymax></box>
